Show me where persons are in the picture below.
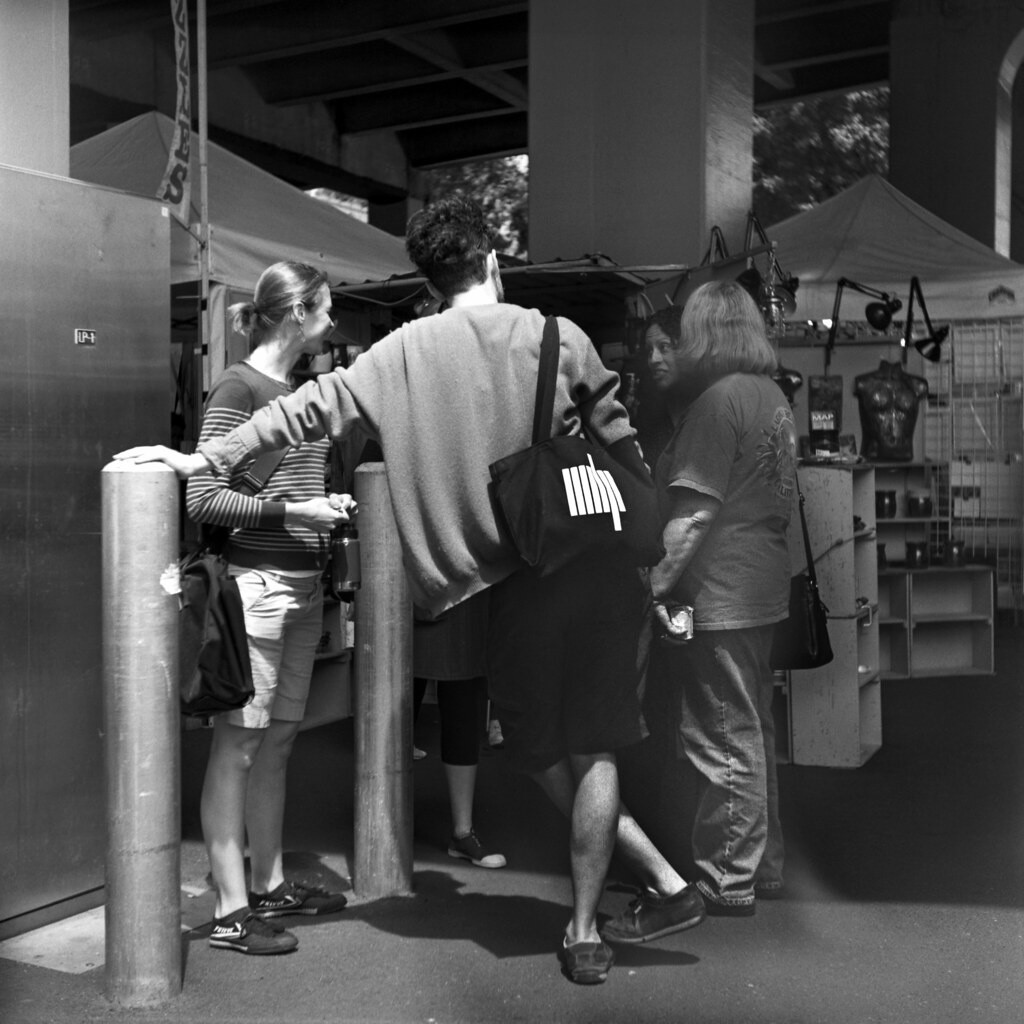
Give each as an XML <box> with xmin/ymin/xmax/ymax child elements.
<box><xmin>348</xmin><ymin>297</ymin><xmax>507</xmax><ymax>869</ymax></box>
<box><xmin>112</xmin><ymin>199</ymin><xmax>705</xmax><ymax>984</ymax></box>
<box><xmin>185</xmin><ymin>262</ymin><xmax>360</xmax><ymax>955</ymax></box>
<box><xmin>649</xmin><ymin>278</ymin><xmax>797</xmax><ymax>916</ymax></box>
<box><xmin>640</xmin><ymin>305</ymin><xmax>689</xmax><ymax>476</ymax></box>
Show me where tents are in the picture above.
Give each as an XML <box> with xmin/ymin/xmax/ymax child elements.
<box><xmin>69</xmin><ymin>109</ymin><xmax>1023</xmax><ymax>391</ymax></box>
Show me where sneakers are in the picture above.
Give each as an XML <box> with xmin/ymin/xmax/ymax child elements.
<box><xmin>249</xmin><ymin>880</ymin><xmax>348</xmax><ymax>917</ymax></box>
<box><xmin>599</xmin><ymin>882</ymin><xmax>707</xmax><ymax>943</ymax></box>
<box><xmin>447</xmin><ymin>827</ymin><xmax>506</xmax><ymax>868</ymax></box>
<box><xmin>208</xmin><ymin>911</ymin><xmax>299</xmax><ymax>955</ymax></box>
<box><xmin>562</xmin><ymin>933</ymin><xmax>613</xmax><ymax>982</ymax></box>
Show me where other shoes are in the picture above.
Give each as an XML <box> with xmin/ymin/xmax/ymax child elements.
<box><xmin>754</xmin><ymin>887</ymin><xmax>786</xmax><ymax>898</ymax></box>
<box><xmin>701</xmin><ymin>892</ymin><xmax>755</xmax><ymax>916</ymax></box>
<box><xmin>413</xmin><ymin>745</ymin><xmax>427</xmax><ymax>759</ymax></box>
<box><xmin>488</xmin><ymin>719</ymin><xmax>504</xmax><ymax>746</ymax></box>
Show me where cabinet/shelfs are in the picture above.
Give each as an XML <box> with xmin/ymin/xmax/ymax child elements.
<box><xmin>789</xmin><ymin>458</ymin><xmax>994</xmax><ymax>769</ymax></box>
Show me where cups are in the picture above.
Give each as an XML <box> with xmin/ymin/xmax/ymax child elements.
<box><xmin>669</xmin><ymin>605</ymin><xmax>694</xmax><ymax>640</ymax></box>
<box><xmin>295</xmin><ymin>339</ymin><xmax>363</xmax><ymax>373</ymax></box>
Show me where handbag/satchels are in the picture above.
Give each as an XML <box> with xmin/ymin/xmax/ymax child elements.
<box><xmin>179</xmin><ymin>552</ymin><xmax>256</xmax><ymax>717</ymax></box>
<box><xmin>771</xmin><ymin>574</ymin><xmax>834</xmax><ymax>670</ymax></box>
<box><xmin>489</xmin><ymin>315</ymin><xmax>667</xmax><ymax>577</ymax></box>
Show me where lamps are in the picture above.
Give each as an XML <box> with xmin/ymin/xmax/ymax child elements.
<box><xmin>743</xmin><ymin>211</ymin><xmax>799</xmax><ymax>313</ymax></box>
<box><xmin>823</xmin><ymin>277</ymin><xmax>902</xmax><ymax>376</ymax></box>
<box><xmin>903</xmin><ymin>275</ymin><xmax>952</xmax><ymax>367</ymax></box>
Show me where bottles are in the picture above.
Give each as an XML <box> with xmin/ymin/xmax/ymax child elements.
<box><xmin>905</xmin><ymin>541</ymin><xmax>929</xmax><ymax>569</ymax></box>
<box><xmin>876</xmin><ymin>543</ymin><xmax>887</xmax><ymax>570</ymax></box>
<box><xmin>332</xmin><ymin>524</ymin><xmax>361</xmax><ymax>600</ymax></box>
<box><xmin>945</xmin><ymin>540</ymin><xmax>964</xmax><ymax>567</ymax></box>
<box><xmin>907</xmin><ymin>487</ymin><xmax>932</xmax><ymax>517</ymax></box>
<box><xmin>875</xmin><ymin>489</ymin><xmax>896</xmax><ymax>519</ymax></box>
